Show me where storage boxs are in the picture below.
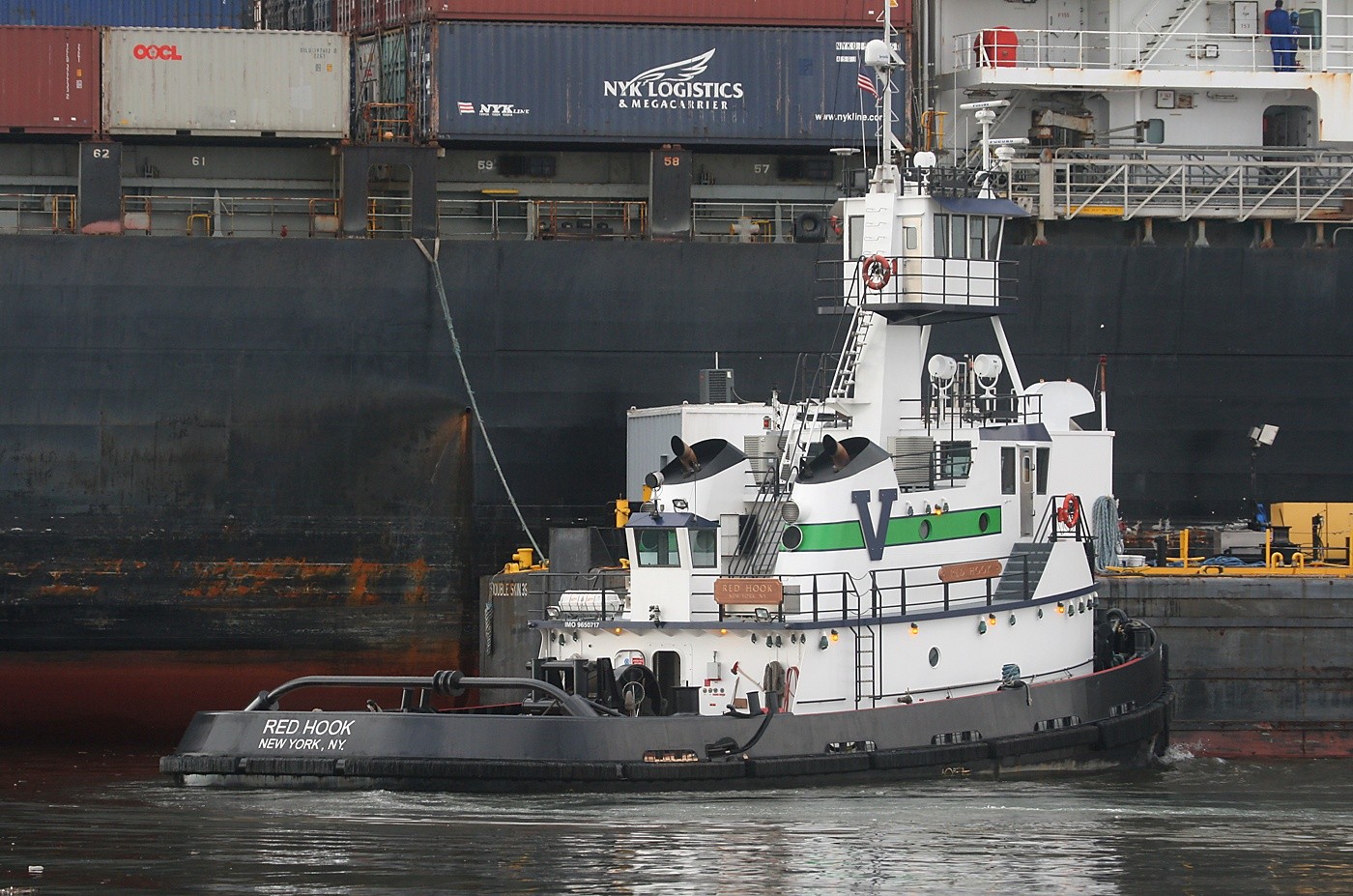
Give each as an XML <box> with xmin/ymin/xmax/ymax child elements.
<box><xmin>0</xmin><ymin>0</ymin><xmax>917</xmax><ymax>151</ymax></box>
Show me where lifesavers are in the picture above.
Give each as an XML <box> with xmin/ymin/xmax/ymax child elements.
<box><xmin>831</xmin><ymin>214</ymin><xmax>844</xmax><ymax>234</ymax></box>
<box><xmin>862</xmin><ymin>253</ymin><xmax>891</xmax><ymax>290</ymax></box>
<box><xmin>1063</xmin><ymin>493</ymin><xmax>1079</xmax><ymax>528</ymax></box>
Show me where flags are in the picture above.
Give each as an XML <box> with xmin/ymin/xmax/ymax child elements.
<box><xmin>856</xmin><ymin>60</ymin><xmax>881</xmax><ymax>104</ymax></box>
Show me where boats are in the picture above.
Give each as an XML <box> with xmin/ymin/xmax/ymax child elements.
<box><xmin>162</xmin><ymin>0</ymin><xmax>1171</xmax><ymax>787</ymax></box>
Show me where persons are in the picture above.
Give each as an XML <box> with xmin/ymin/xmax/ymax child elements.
<box><xmin>1266</xmin><ymin>0</ymin><xmax>1300</xmax><ymax>72</ymax></box>
